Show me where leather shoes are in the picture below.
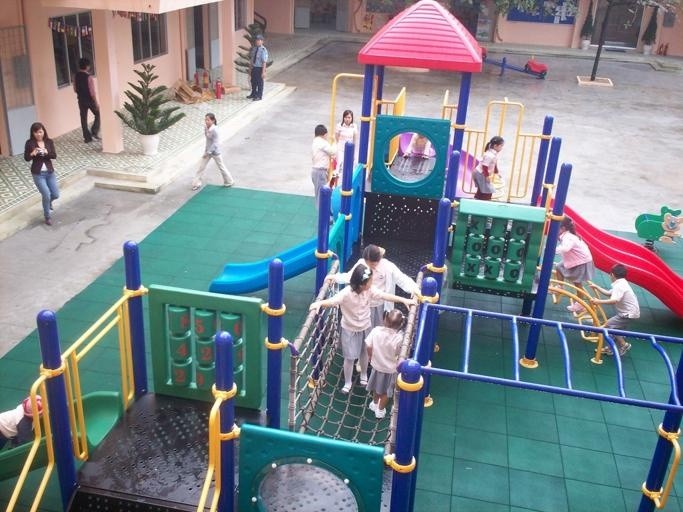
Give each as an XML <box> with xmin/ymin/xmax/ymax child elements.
<box><xmin>46</xmin><ymin>217</ymin><xmax>52</xmax><ymax>225</ymax></box>
<box><xmin>247</xmin><ymin>94</ymin><xmax>262</xmax><ymax>101</ymax></box>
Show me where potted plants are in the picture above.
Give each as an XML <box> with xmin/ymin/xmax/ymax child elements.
<box><xmin>233</xmin><ymin>12</ymin><xmax>275</xmax><ymax>90</ymax></box>
<box><xmin>111</xmin><ymin>58</ymin><xmax>187</xmax><ymax>154</ymax></box>
<box><xmin>579</xmin><ymin>0</ymin><xmax>595</xmax><ymax>51</ymax></box>
<box><xmin>639</xmin><ymin>4</ymin><xmax>662</xmax><ymax>56</ymax></box>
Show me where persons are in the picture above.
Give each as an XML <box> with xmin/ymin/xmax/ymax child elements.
<box><xmin>364</xmin><ymin>309</ymin><xmax>408</xmax><ymax>420</ymax></box>
<box><xmin>191</xmin><ymin>113</ymin><xmax>234</xmax><ymax>191</ymax></box>
<box><xmin>0</xmin><ymin>394</ymin><xmax>44</xmax><ymax>452</ymax></box>
<box><xmin>24</xmin><ymin>123</ymin><xmax>60</xmax><ymax>225</ymax></box>
<box><xmin>309</xmin><ymin>263</ymin><xmax>419</xmax><ymax>395</ymax></box>
<box><xmin>402</xmin><ymin>132</ymin><xmax>433</xmax><ymax>160</ymax></box>
<box><xmin>589</xmin><ymin>263</ymin><xmax>640</xmax><ymax>357</ymax></box>
<box><xmin>246</xmin><ymin>34</ymin><xmax>269</xmax><ymax>101</ymax></box>
<box><xmin>554</xmin><ymin>215</ymin><xmax>594</xmax><ymax>312</ymax></box>
<box><xmin>335</xmin><ymin>110</ymin><xmax>360</xmax><ymax>166</ymax></box>
<box><xmin>310</xmin><ymin>124</ymin><xmax>336</xmax><ymax>224</ymax></box>
<box><xmin>73</xmin><ymin>58</ymin><xmax>101</xmax><ymax>143</ymax></box>
<box><xmin>324</xmin><ymin>244</ymin><xmax>419</xmax><ymax>372</ymax></box>
<box><xmin>471</xmin><ymin>135</ymin><xmax>504</xmax><ymax>201</ymax></box>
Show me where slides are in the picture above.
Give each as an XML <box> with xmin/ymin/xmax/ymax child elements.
<box><xmin>1</xmin><ymin>390</ymin><xmax>123</xmax><ymax>482</ymax></box>
<box><xmin>208</xmin><ymin>237</ymin><xmax>317</xmax><ymax>293</ymax></box>
<box><xmin>535</xmin><ymin>194</ymin><xmax>683</xmax><ymax>318</ymax></box>
<box><xmin>399</xmin><ymin>132</ymin><xmax>483</xmax><ymax>200</ymax></box>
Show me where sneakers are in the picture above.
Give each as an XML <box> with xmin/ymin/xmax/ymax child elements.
<box><xmin>595</xmin><ymin>347</ymin><xmax>613</xmax><ymax>356</ymax></box>
<box><xmin>618</xmin><ymin>343</ymin><xmax>631</xmax><ymax>355</ymax></box>
<box><xmin>341</xmin><ymin>384</ymin><xmax>351</xmax><ymax>395</ymax></box>
<box><xmin>369</xmin><ymin>399</ymin><xmax>387</xmax><ymax>418</ymax></box>
<box><xmin>356</xmin><ymin>360</ymin><xmax>361</xmax><ymax>372</ymax></box>
<box><xmin>360</xmin><ymin>379</ymin><xmax>368</xmax><ymax>387</ymax></box>
<box><xmin>567</xmin><ymin>301</ymin><xmax>584</xmax><ymax>312</ymax></box>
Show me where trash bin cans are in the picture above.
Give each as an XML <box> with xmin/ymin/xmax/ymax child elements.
<box><xmin>294</xmin><ymin>5</ymin><xmax>310</xmax><ymax>29</ymax></box>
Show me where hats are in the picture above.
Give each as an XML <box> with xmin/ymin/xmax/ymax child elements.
<box><xmin>24</xmin><ymin>395</ymin><xmax>44</xmax><ymax>417</ymax></box>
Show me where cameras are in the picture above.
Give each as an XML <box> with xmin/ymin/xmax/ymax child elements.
<box><xmin>37</xmin><ymin>149</ymin><xmax>45</xmax><ymax>156</ymax></box>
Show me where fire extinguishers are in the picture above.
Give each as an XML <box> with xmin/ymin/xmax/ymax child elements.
<box><xmin>659</xmin><ymin>43</ymin><xmax>670</xmax><ymax>56</ymax></box>
<box><xmin>215</xmin><ymin>77</ymin><xmax>223</xmax><ymax>99</ymax></box>
<box><xmin>194</xmin><ymin>70</ymin><xmax>200</xmax><ymax>87</ymax></box>
<box><xmin>202</xmin><ymin>69</ymin><xmax>211</xmax><ymax>88</ymax></box>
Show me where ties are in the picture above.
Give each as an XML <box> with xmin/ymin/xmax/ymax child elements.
<box><xmin>253</xmin><ymin>48</ymin><xmax>258</xmax><ymax>63</ymax></box>
